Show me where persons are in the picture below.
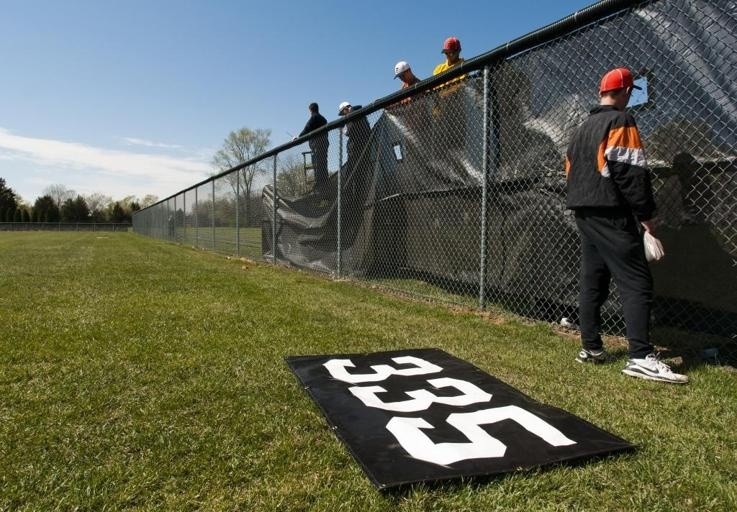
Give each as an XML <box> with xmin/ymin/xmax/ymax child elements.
<box><xmin>292</xmin><ymin>102</ymin><xmax>329</xmax><ymax>189</ymax></box>
<box><xmin>338</xmin><ymin>101</ymin><xmax>372</xmax><ymax>171</ymax></box>
<box><xmin>373</xmin><ymin>61</ymin><xmax>425</xmax><ymax>124</ymax></box>
<box><xmin>168</xmin><ymin>215</ymin><xmax>175</xmax><ymax>238</ymax></box>
<box><xmin>429</xmin><ymin>36</ymin><xmax>470</xmax><ymax>136</ymax></box>
<box><xmin>565</xmin><ymin>66</ymin><xmax>692</xmax><ymax>384</ymax></box>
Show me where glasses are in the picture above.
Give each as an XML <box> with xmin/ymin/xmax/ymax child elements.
<box><xmin>445</xmin><ymin>52</ymin><xmax>457</xmax><ymax>57</ymax></box>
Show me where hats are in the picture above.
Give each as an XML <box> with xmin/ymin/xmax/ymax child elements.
<box><xmin>441</xmin><ymin>37</ymin><xmax>462</xmax><ymax>54</ymax></box>
<box><xmin>600</xmin><ymin>68</ymin><xmax>642</xmax><ymax>92</ymax></box>
<box><xmin>394</xmin><ymin>61</ymin><xmax>410</xmax><ymax>78</ymax></box>
<box><xmin>339</xmin><ymin>102</ymin><xmax>350</xmax><ymax>114</ymax></box>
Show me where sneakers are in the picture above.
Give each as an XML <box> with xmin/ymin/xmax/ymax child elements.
<box><xmin>621</xmin><ymin>354</ymin><xmax>689</xmax><ymax>384</ymax></box>
<box><xmin>575</xmin><ymin>348</ymin><xmax>618</xmax><ymax>365</ymax></box>
